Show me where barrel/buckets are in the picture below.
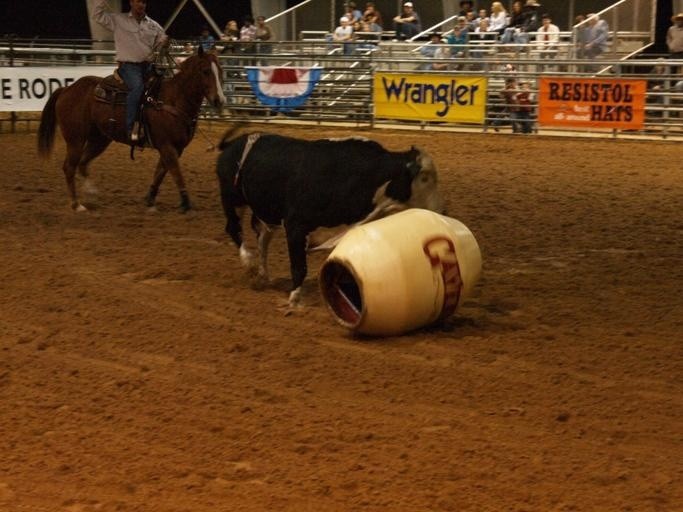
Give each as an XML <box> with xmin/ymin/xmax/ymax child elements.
<box><xmin>316</xmin><ymin>207</ymin><xmax>482</xmax><ymax>338</ymax></box>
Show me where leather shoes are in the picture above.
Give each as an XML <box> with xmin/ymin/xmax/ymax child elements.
<box><xmin>127</xmin><ymin>130</ymin><xmax>144</xmax><ymax>138</ymax></box>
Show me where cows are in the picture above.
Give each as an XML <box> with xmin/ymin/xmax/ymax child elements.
<box><xmin>212</xmin><ymin>116</ymin><xmax>449</xmax><ymax>309</ymax></box>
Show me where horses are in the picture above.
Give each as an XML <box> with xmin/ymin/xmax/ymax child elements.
<box><xmin>33</xmin><ymin>40</ymin><xmax>228</xmax><ymax>216</ymax></box>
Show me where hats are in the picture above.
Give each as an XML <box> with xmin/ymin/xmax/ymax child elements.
<box><xmin>343</xmin><ymin>2</ymin><xmax>413</xmax><ymax>8</ymax></box>
<box><xmin>524</xmin><ymin>1</ymin><xmax>540</xmax><ymax>8</ymax></box>
<box><xmin>671</xmin><ymin>12</ymin><xmax>683</xmax><ymax>24</ymax></box>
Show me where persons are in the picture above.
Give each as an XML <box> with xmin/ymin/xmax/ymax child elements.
<box><xmin>666</xmin><ymin>13</ymin><xmax>683</xmax><ymax>85</ymax></box>
<box><xmin>393</xmin><ymin>3</ymin><xmax>421</xmax><ymax>41</ymax></box>
<box><xmin>573</xmin><ymin>13</ymin><xmax>609</xmax><ymax>58</ymax></box>
<box><xmin>419</xmin><ymin>1</ymin><xmax>542</xmax><ymax>71</ymax></box>
<box><xmin>89</xmin><ymin>1</ymin><xmax>169</xmax><ymax>144</ymax></box>
<box><xmin>536</xmin><ymin>13</ymin><xmax>560</xmax><ymax>68</ymax></box>
<box><xmin>174</xmin><ymin>15</ymin><xmax>275</xmax><ymax>65</ymax></box>
<box><xmin>327</xmin><ymin>2</ymin><xmax>383</xmax><ymax>52</ymax></box>
<box><xmin>492</xmin><ymin>78</ymin><xmax>536</xmax><ymax>135</ymax></box>
